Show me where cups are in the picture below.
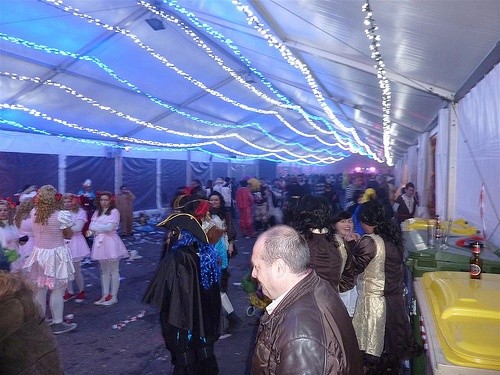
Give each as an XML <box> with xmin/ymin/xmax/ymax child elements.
<box><xmin>427</xmin><ymin>226</ymin><xmax>433</xmax><ymax>247</ymax></box>
<box><xmin>441</xmin><ymin>222</ymin><xmax>448</xmax><ymax>244</ymax></box>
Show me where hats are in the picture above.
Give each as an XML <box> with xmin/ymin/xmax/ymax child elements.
<box><xmin>157</xmin><ymin>194</ymin><xmax>223</xmax><ymax>245</ymax></box>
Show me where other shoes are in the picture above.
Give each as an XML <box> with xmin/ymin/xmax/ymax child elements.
<box><xmin>63</xmin><ymin>290</ymin><xmax>87</xmax><ymax>303</ymax></box>
<box><xmin>92</xmin><ymin>294</ymin><xmax>119</xmax><ymax>306</ymax></box>
<box><xmin>47</xmin><ymin>320</ymin><xmax>77</xmax><ymax>334</ymax></box>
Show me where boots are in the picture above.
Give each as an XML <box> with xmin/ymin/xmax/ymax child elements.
<box><xmin>224</xmin><ymin>310</ymin><xmax>242</xmax><ymax>334</ymax></box>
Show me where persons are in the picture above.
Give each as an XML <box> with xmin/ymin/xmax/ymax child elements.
<box><xmin>338</xmin><ymin>200</ymin><xmax>419</xmax><ymax>375</ymax></box>
<box><xmin>173</xmin><ymin>171</ymin><xmax>419</xmax><ymax>333</ymax></box>
<box><xmin>250</xmin><ymin>226</ymin><xmax>364</xmax><ymax>375</ymax></box>
<box><xmin>0</xmin><ymin>244</ymin><xmax>62</xmax><ymax>375</ymax></box>
<box><xmin>140</xmin><ymin>195</ymin><xmax>222</xmax><ymax>375</ymax></box>
<box><xmin>0</xmin><ymin>179</ymin><xmax>135</xmax><ymax>333</ymax></box>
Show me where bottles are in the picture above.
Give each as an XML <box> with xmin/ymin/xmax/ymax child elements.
<box><xmin>470</xmin><ymin>240</ymin><xmax>482</xmax><ymax>278</ymax></box>
<box><xmin>435</xmin><ymin>215</ymin><xmax>441</xmax><ymax>237</ymax></box>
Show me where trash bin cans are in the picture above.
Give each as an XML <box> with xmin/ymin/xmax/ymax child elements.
<box><xmin>401</xmin><ymin>218</ymin><xmax>500</xmax><ymax>374</ymax></box>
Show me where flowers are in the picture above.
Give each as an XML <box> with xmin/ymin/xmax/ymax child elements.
<box><xmin>54</xmin><ymin>192</ymin><xmax>63</xmax><ymax>201</ymax></box>
<box><xmin>33</xmin><ymin>189</ymin><xmax>43</xmax><ymax>204</ymax></box>
<box><xmin>110</xmin><ymin>194</ymin><xmax>116</xmax><ymax>202</ymax></box>
<box><xmin>6</xmin><ymin>195</ymin><xmax>17</xmax><ymax>209</ymax></box>
<box><xmin>0</xmin><ymin>196</ymin><xmax>4</xmax><ymax>203</ymax></box>
<box><xmin>95</xmin><ymin>190</ymin><xmax>101</xmax><ymax>198</ymax></box>
<box><xmin>71</xmin><ymin>194</ymin><xmax>83</xmax><ymax>205</ymax></box>
